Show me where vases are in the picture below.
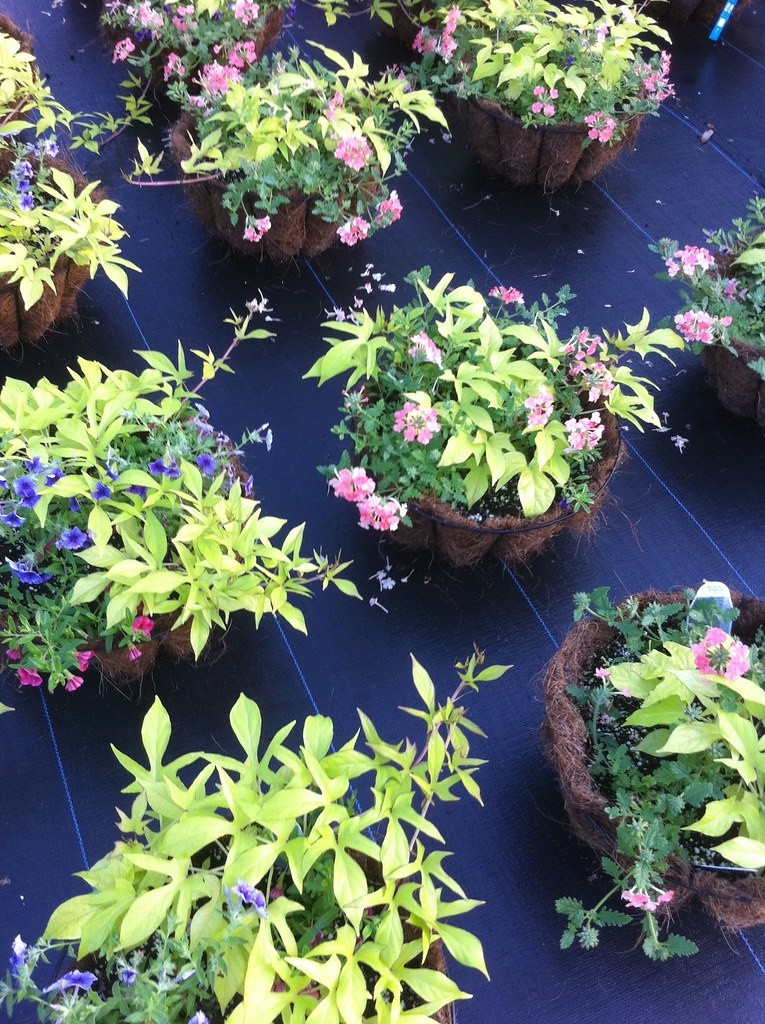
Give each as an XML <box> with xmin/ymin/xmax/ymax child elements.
<box><xmin>384</xmin><ymin>394</ymin><xmax>626</xmax><ymax>571</ymax></box>
<box><xmin>96</xmin><ymin>0</ymin><xmax>286</xmax><ymax>90</ymax></box>
<box><xmin>701</xmin><ymin>245</ymin><xmax>765</xmax><ymax>423</ymax></box>
<box><xmin>0</xmin><ymin>13</ymin><xmax>38</xmax><ymax>173</ymax></box>
<box><xmin>451</xmin><ymin>73</ymin><xmax>647</xmax><ymax>190</ymax></box>
<box><xmin>0</xmin><ymin>145</ymin><xmax>110</xmax><ymax>347</ymax></box>
<box><xmin>77</xmin><ymin>408</ymin><xmax>255</xmax><ymax>684</ymax></box>
<box><xmin>540</xmin><ymin>585</ymin><xmax>765</xmax><ymax>935</ymax></box>
<box><xmin>169</xmin><ymin>110</ymin><xmax>384</xmax><ymax>256</ymax></box>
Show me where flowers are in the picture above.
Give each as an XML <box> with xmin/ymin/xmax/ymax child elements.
<box><xmin>0</xmin><ymin>0</ymin><xmax>765</xmax><ymax>1024</ymax></box>
